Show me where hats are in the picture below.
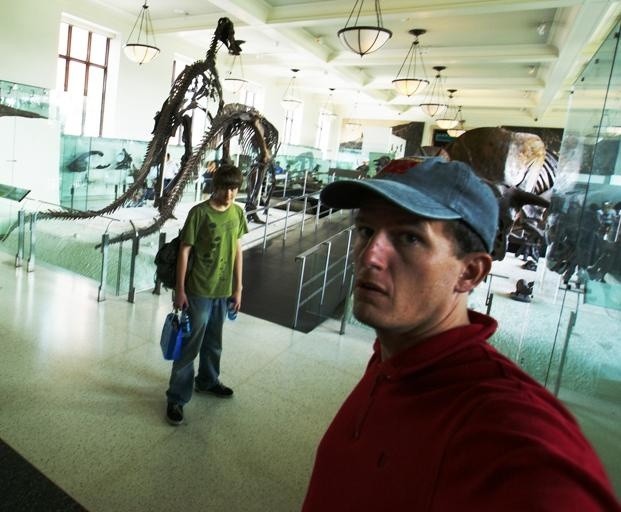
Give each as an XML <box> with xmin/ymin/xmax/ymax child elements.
<box><xmin>319</xmin><ymin>156</ymin><xmax>499</xmax><ymax>255</ymax></box>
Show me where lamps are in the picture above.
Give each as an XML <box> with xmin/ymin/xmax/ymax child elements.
<box><xmin>122</xmin><ymin>0</ymin><xmax>160</xmax><ymax>65</ymax></box>
<box><xmin>338</xmin><ymin>1</ymin><xmax>466</xmax><ymax>140</ymax></box>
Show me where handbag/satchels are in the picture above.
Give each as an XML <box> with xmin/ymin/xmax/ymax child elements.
<box><xmin>161</xmin><ymin>313</ymin><xmax>184</xmax><ymax>360</ymax></box>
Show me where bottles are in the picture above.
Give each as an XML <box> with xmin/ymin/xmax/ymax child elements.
<box><xmin>181</xmin><ymin>310</ymin><xmax>192</xmax><ymax>336</ymax></box>
<box><xmin>227</xmin><ymin>295</ymin><xmax>238</xmax><ymax>321</ymax></box>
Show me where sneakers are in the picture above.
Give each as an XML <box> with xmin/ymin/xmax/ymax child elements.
<box><xmin>166</xmin><ymin>401</ymin><xmax>184</xmax><ymax>425</ymax></box>
<box><xmin>195</xmin><ymin>381</ymin><xmax>233</xmax><ymax>396</ymax></box>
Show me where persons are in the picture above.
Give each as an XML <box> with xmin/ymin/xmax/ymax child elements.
<box><xmin>146</xmin><ymin>152</ymin><xmax>290</xmax><ymax>198</ymax></box>
<box><xmin>302</xmin><ymin>155</ymin><xmax>621</xmax><ymax>512</ymax></box>
<box><xmin>165</xmin><ymin>165</ymin><xmax>250</xmax><ymax>426</ymax></box>
<box><xmin>567</xmin><ymin>195</ymin><xmax>621</xmax><ymax>266</ymax></box>
